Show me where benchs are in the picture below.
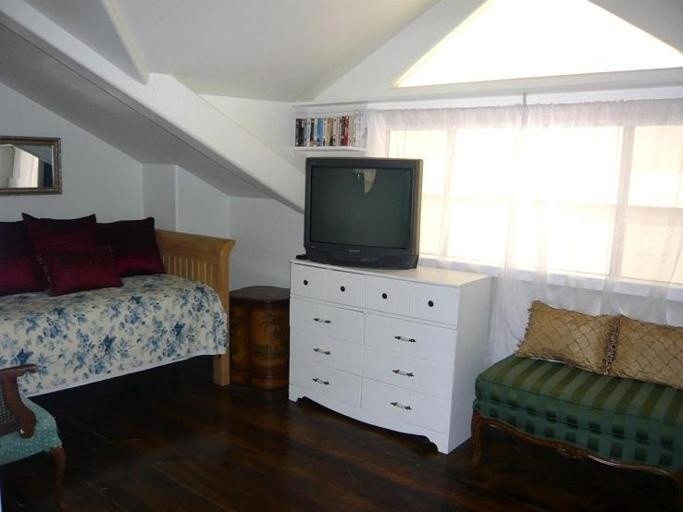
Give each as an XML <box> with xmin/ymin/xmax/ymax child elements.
<box><xmin>468</xmin><ymin>353</ymin><xmax>682</xmax><ymax>486</ymax></box>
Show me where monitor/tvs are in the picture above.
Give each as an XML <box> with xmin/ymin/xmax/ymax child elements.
<box><xmin>304</xmin><ymin>156</ymin><xmax>423</xmax><ymax>270</ymax></box>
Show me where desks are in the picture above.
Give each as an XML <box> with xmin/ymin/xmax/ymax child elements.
<box><xmin>0</xmin><ymin>390</ymin><xmax>68</xmax><ymax>511</ymax></box>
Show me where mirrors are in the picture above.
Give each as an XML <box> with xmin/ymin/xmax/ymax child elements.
<box><xmin>0</xmin><ymin>135</ymin><xmax>62</xmax><ymax>197</ymax></box>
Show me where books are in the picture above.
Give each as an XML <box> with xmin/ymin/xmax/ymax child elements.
<box><xmin>294</xmin><ymin>114</ymin><xmax>366</xmax><ymax>148</ymax></box>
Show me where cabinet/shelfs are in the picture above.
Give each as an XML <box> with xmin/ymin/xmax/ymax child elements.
<box><xmin>286</xmin><ymin>258</ymin><xmax>495</xmax><ymax>455</ymax></box>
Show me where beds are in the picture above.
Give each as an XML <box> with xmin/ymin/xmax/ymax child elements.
<box><xmin>0</xmin><ymin>228</ymin><xmax>235</xmax><ymax>420</ymax></box>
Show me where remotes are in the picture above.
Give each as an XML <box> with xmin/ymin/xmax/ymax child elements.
<box><xmin>296</xmin><ymin>254</ymin><xmax>307</xmax><ymax>260</ymax></box>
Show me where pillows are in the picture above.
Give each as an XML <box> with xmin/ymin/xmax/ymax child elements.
<box><xmin>0</xmin><ymin>211</ymin><xmax>165</xmax><ymax>297</ymax></box>
<box><xmin>513</xmin><ymin>299</ymin><xmax>682</xmax><ymax>391</ymax></box>
